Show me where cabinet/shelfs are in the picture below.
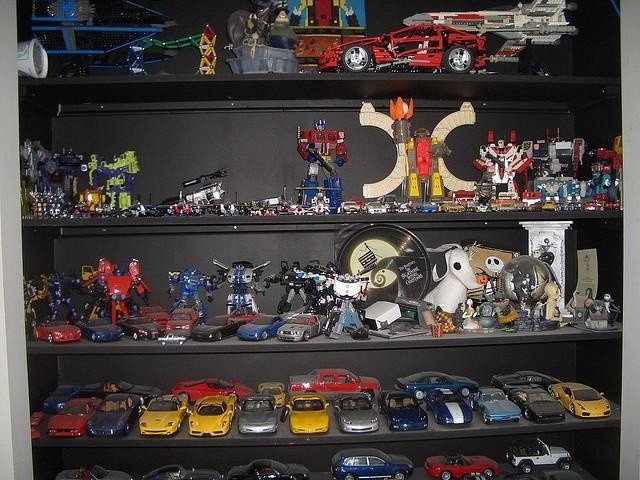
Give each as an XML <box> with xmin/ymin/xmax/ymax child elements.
<box><xmin>16</xmin><ymin>1</ymin><xmax>625</xmax><ymax>480</ymax></box>
